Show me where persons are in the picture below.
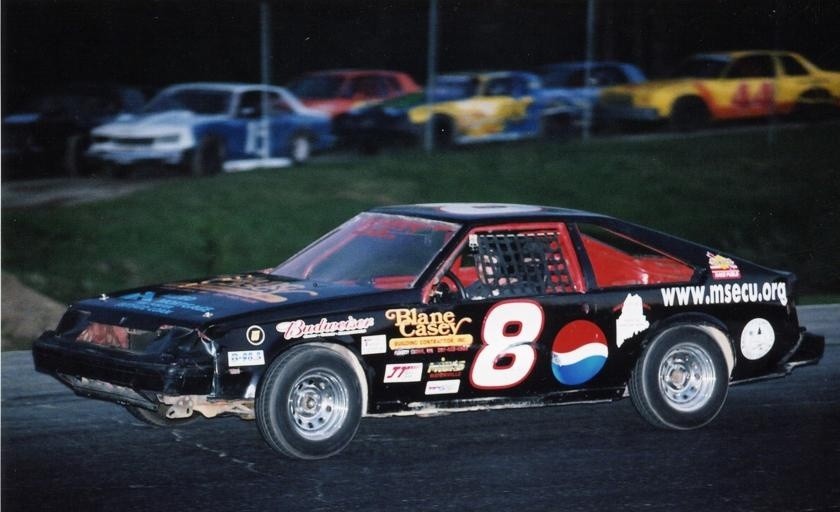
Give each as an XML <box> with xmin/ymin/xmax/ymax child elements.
<box><xmin>449</xmin><ymin>250</ymin><xmax>538</xmax><ymax>303</ymax></box>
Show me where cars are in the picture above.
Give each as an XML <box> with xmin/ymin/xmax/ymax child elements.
<box><xmin>32</xmin><ymin>200</ymin><xmax>825</xmax><ymax>462</ymax></box>
